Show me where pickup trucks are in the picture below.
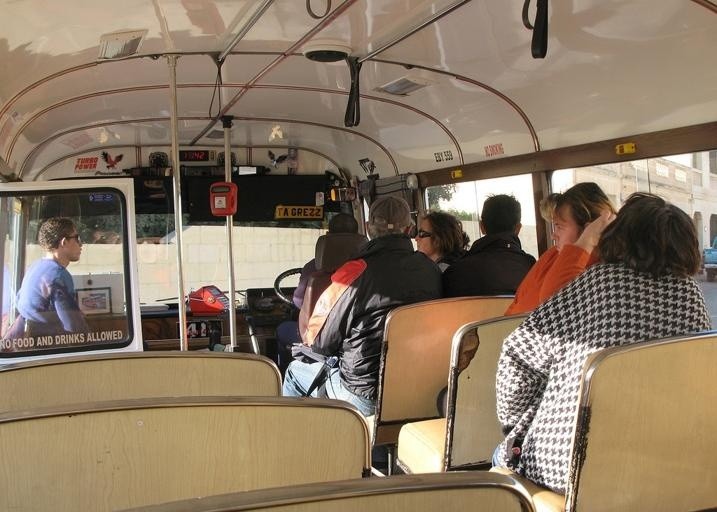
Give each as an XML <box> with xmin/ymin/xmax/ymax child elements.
<box><xmin>701</xmin><ymin>236</ymin><xmax>717</xmax><ymax>282</ymax></box>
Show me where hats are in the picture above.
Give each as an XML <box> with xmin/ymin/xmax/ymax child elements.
<box><xmin>368</xmin><ymin>195</ymin><xmax>416</xmax><ymax>230</ymax></box>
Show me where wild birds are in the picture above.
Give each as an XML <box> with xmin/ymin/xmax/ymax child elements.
<box><xmin>100</xmin><ymin>152</ymin><xmax>124</xmax><ymax>170</ymax></box>
<box><xmin>268</xmin><ymin>151</ymin><xmax>289</xmax><ymax>169</ymax></box>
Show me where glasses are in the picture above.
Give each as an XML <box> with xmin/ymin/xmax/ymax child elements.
<box><xmin>418</xmin><ymin>229</ymin><xmax>432</xmax><ymax>238</ymax></box>
<box><xmin>66</xmin><ymin>234</ymin><xmax>81</xmax><ymax>241</ymax></box>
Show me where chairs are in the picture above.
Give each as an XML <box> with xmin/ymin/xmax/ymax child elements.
<box><xmin>393</xmin><ymin>310</ymin><xmax>531</xmax><ymax>475</ymax></box>
<box><xmin>354</xmin><ymin>293</ymin><xmax>519</xmax><ymax>475</ymax></box>
<box><xmin>1</xmin><ymin>395</ymin><xmax>374</xmax><ymax>511</ymax></box>
<box><xmin>1</xmin><ymin>351</ymin><xmax>284</xmax><ymax>412</ymax></box>
<box><xmin>484</xmin><ymin>329</ymin><xmax>717</xmax><ymax>512</ymax></box>
<box><xmin>110</xmin><ymin>469</ymin><xmax>536</xmax><ymax>512</ymax></box>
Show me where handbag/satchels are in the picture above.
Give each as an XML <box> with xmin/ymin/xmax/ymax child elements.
<box><xmin>290</xmin><ymin>341</ymin><xmax>340</xmax><ymax>368</ymax></box>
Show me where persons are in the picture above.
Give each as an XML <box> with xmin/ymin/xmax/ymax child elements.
<box><xmin>13</xmin><ymin>216</ymin><xmax>95</xmax><ymax>350</ymax></box>
<box><xmin>491</xmin><ymin>182</ymin><xmax>717</xmax><ymax>496</ymax></box>
<box><xmin>275</xmin><ymin>192</ymin><xmax>536</xmax><ymax>416</ymax></box>
